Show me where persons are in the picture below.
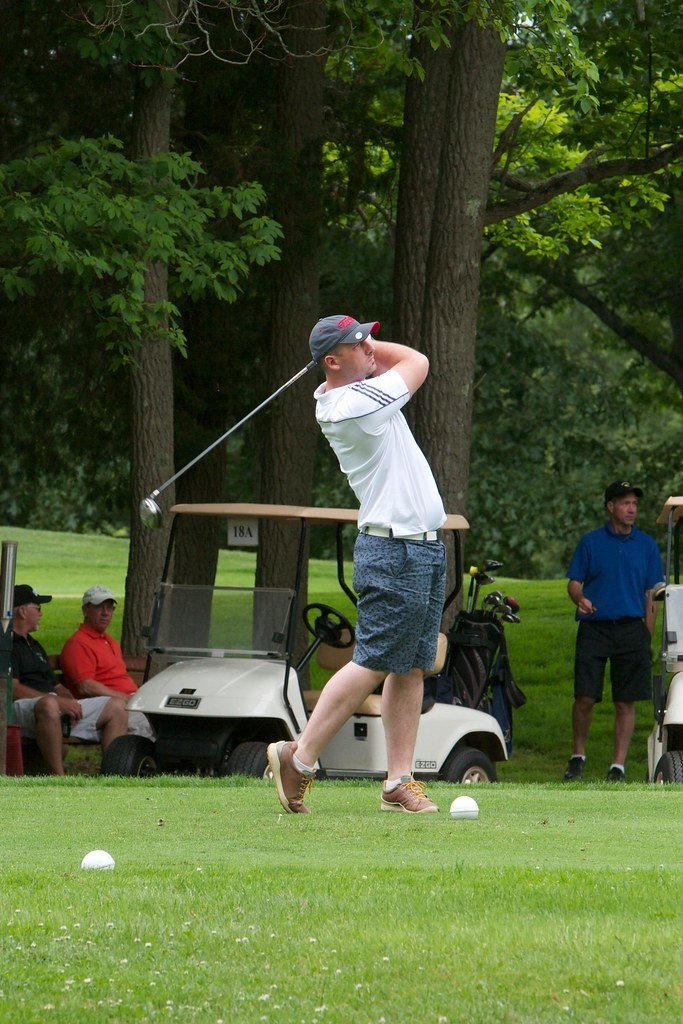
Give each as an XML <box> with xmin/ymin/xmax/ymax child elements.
<box><xmin>267</xmin><ymin>315</ymin><xmax>446</xmax><ymax>814</ymax></box>
<box><xmin>564</xmin><ymin>481</ymin><xmax>666</xmax><ymax>782</ymax></box>
<box><xmin>0</xmin><ymin>584</ymin><xmax>152</xmax><ymax>777</ymax></box>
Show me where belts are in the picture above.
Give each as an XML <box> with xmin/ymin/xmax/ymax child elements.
<box><xmin>602</xmin><ymin>616</ymin><xmax>642</xmax><ymax>624</ymax></box>
<box><xmin>361</xmin><ymin>528</ymin><xmax>437</xmax><ymax>541</ymax></box>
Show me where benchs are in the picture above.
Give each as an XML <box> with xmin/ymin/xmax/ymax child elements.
<box><xmin>302</xmin><ymin>628</ymin><xmax>448</xmax><ymax>715</ymax></box>
<box><xmin>47</xmin><ymin>655</ymin><xmax>159</xmax><ymax>742</ymax></box>
<box><xmin>665</xmin><ymin>584</ymin><xmax>683</xmax><ymax>673</ymax></box>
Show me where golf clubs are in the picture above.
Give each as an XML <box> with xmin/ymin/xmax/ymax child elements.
<box><xmin>468</xmin><ymin>558</ymin><xmax>522</xmax><ymax>625</ymax></box>
<box><xmin>140</xmin><ymin>359</ymin><xmax>316</xmax><ymax>529</ymax></box>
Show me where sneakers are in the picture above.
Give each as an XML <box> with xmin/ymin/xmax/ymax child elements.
<box><xmin>381</xmin><ymin>779</ymin><xmax>442</xmax><ymax>812</ymax></box>
<box><xmin>608</xmin><ymin>767</ymin><xmax>625</xmax><ymax>782</ymax></box>
<box><xmin>563</xmin><ymin>756</ymin><xmax>585</xmax><ymax>781</ymax></box>
<box><xmin>267</xmin><ymin>741</ymin><xmax>316</xmax><ymax>813</ymax></box>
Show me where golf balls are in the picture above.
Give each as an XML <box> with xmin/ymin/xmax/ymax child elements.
<box><xmin>81</xmin><ymin>850</ymin><xmax>115</xmax><ymax>871</ymax></box>
<box><xmin>449</xmin><ymin>796</ymin><xmax>481</xmax><ymax>821</ymax></box>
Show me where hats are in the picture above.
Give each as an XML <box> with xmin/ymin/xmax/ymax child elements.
<box><xmin>83</xmin><ymin>586</ymin><xmax>118</xmax><ymax>606</ymax></box>
<box><xmin>309</xmin><ymin>315</ymin><xmax>381</xmax><ymax>360</ymax></box>
<box><xmin>605</xmin><ymin>480</ymin><xmax>644</xmax><ymax>497</ymax></box>
<box><xmin>15</xmin><ymin>584</ymin><xmax>53</xmax><ymax>604</ymax></box>
